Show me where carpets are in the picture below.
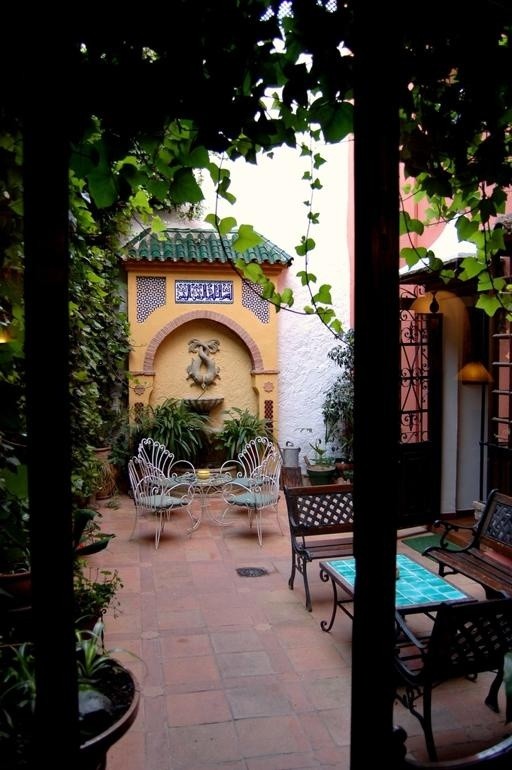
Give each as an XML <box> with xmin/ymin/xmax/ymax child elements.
<box><xmin>401</xmin><ymin>533</ymin><xmax>464</xmax><ymax>563</ymax></box>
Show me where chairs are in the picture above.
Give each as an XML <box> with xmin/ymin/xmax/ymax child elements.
<box><xmin>126</xmin><ymin>436</ymin><xmax>285</xmax><ymax>552</ymax></box>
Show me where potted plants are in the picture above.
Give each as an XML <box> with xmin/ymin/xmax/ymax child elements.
<box><xmin>1</xmin><ymin>462</ymin><xmax>142</xmax><ymax>770</ymax></box>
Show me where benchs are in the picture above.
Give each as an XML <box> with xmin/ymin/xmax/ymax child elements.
<box><xmin>422</xmin><ymin>488</ymin><xmax>510</xmax><ymax>601</ymax></box>
<box><xmin>377</xmin><ymin>595</ymin><xmax>512</xmax><ymax>763</ymax></box>
<box><xmin>284</xmin><ymin>482</ymin><xmax>356</xmax><ymax>610</ymax></box>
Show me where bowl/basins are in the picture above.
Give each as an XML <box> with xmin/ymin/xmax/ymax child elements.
<box><xmin>197</xmin><ymin>470</ymin><xmax>211</xmax><ymax>479</ymax></box>
